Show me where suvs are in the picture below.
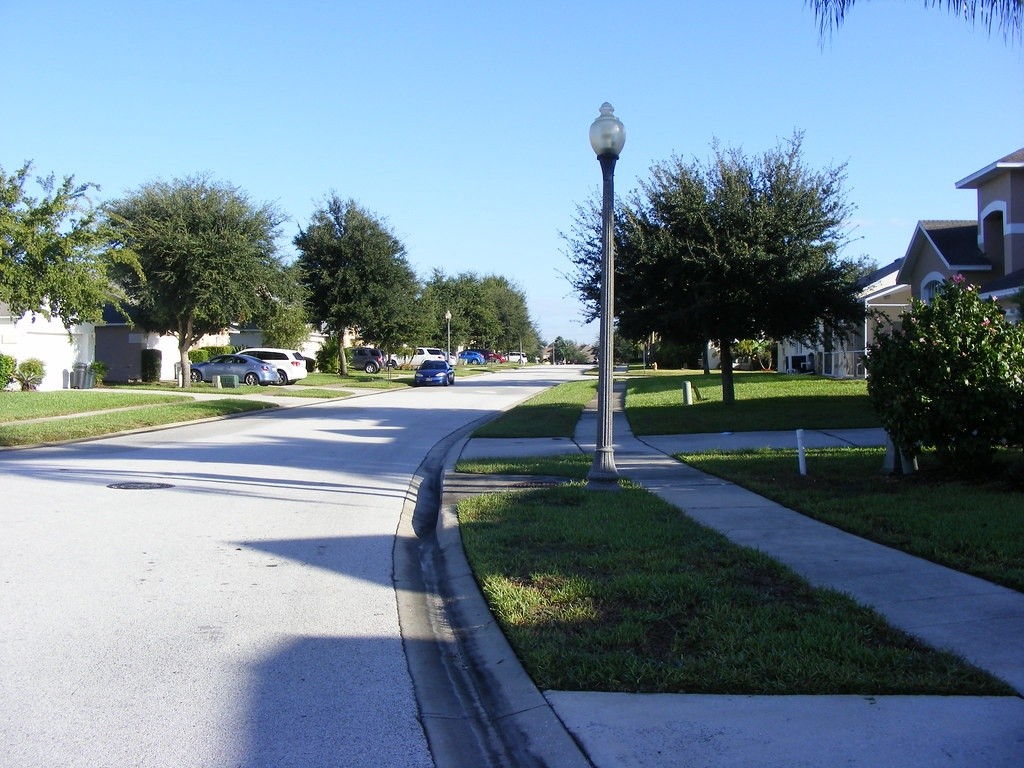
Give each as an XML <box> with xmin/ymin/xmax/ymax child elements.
<box><xmin>234</xmin><ymin>348</ymin><xmax>308</xmax><ymax>386</ymax></box>
<box><xmin>343</xmin><ymin>346</ymin><xmax>384</xmax><ymax>374</ymax></box>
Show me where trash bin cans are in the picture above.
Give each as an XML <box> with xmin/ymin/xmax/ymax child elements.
<box><xmin>72</xmin><ymin>362</ymin><xmax>87</xmax><ymax>389</ymax></box>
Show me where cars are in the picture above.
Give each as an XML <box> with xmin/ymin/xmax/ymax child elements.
<box><xmin>385</xmin><ymin>346</ymin><xmax>445</xmax><ymax>372</ymax></box>
<box><xmin>414</xmin><ymin>360</ymin><xmax>454</xmax><ymax>387</ymax></box>
<box><xmin>189</xmin><ymin>353</ymin><xmax>279</xmax><ymax>386</ymax></box>
<box><xmin>446</xmin><ymin>348</ymin><xmax>528</xmax><ymax>367</ymax></box>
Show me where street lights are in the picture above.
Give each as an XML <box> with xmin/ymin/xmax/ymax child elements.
<box><xmin>589</xmin><ymin>101</ymin><xmax>627</xmax><ymax>492</ymax></box>
<box><xmin>445</xmin><ymin>310</ymin><xmax>452</xmax><ymax>366</ymax></box>
